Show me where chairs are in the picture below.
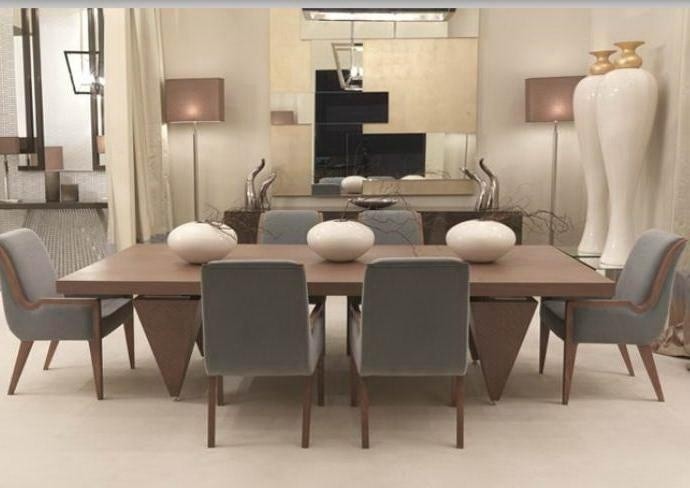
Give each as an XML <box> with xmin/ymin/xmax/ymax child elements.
<box><xmin>200</xmin><ymin>260</ymin><xmax>327</xmax><ymax>449</ymax></box>
<box><xmin>348</xmin><ymin>258</ymin><xmax>469</xmax><ymax>449</ymax></box>
<box><xmin>256</xmin><ymin>210</ymin><xmax>324</xmax><ymax>245</ymax></box>
<box><xmin>540</xmin><ymin>230</ymin><xmax>687</xmax><ymax>406</ymax></box>
<box><xmin>0</xmin><ymin>228</ymin><xmax>135</xmax><ymax>401</ymax></box>
<box><xmin>358</xmin><ymin>210</ymin><xmax>424</xmax><ymax>243</ymax></box>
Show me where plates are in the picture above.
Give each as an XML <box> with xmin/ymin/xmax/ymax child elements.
<box><xmin>352</xmin><ymin>200</ymin><xmax>395</xmax><ymax>211</ymax></box>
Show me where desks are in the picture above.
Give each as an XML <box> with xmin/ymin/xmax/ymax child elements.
<box><xmin>55</xmin><ymin>244</ymin><xmax>617</xmax><ymax>403</ymax></box>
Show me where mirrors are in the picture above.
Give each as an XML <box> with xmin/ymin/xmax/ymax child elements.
<box><xmin>12</xmin><ymin>7</ymin><xmax>107</xmax><ymax>173</ymax></box>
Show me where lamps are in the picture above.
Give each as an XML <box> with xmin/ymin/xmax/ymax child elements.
<box><xmin>524</xmin><ymin>75</ymin><xmax>586</xmax><ymax>246</ymax></box>
<box><xmin>0</xmin><ymin>136</ymin><xmax>35</xmax><ymax>203</ymax></box>
<box><xmin>30</xmin><ymin>147</ymin><xmax>64</xmax><ymax>170</ymax></box>
<box><xmin>159</xmin><ymin>77</ymin><xmax>225</xmax><ymax>223</ymax></box>
<box><xmin>302</xmin><ymin>7</ymin><xmax>456</xmax><ymax>22</ymax></box>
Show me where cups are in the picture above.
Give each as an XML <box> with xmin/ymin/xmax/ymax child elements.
<box><xmin>61</xmin><ymin>184</ymin><xmax>78</xmax><ymax>202</ymax></box>
<box><xmin>44</xmin><ymin>171</ymin><xmax>60</xmax><ymax>202</ymax></box>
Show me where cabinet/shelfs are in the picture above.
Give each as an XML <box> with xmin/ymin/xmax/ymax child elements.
<box><xmin>224</xmin><ymin>195</ymin><xmax>525</xmax><ymax>247</ymax></box>
